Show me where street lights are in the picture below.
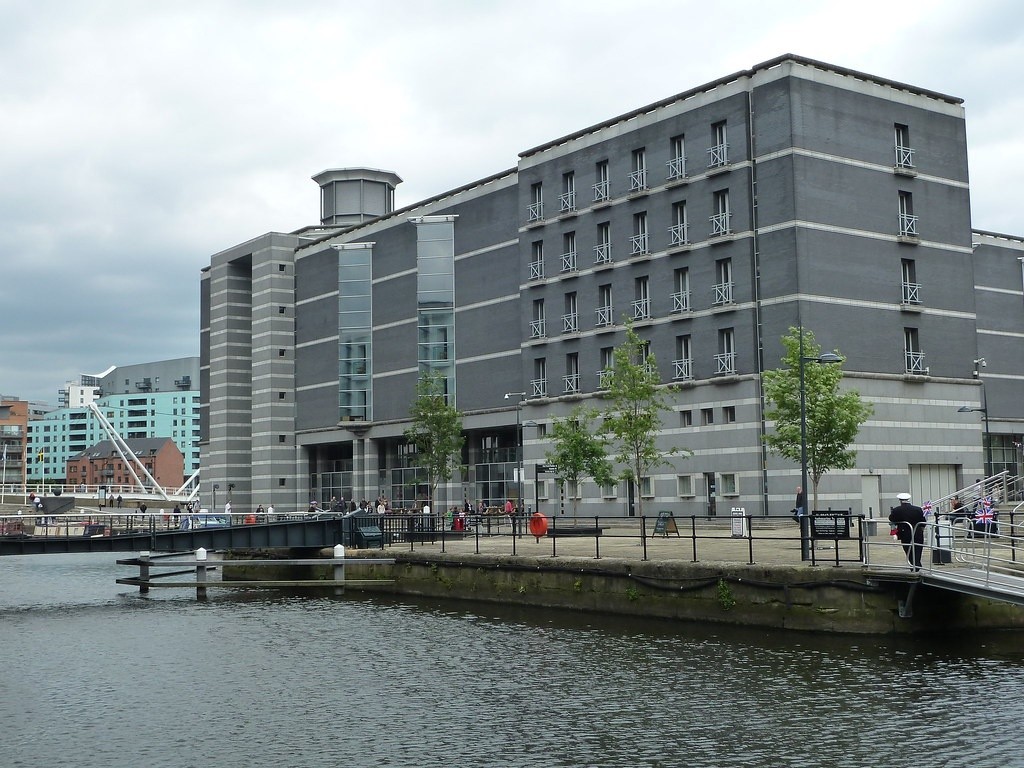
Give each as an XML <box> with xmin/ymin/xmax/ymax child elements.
<box><xmin>957</xmin><ymin>406</ymin><xmax>994</xmax><ymax>505</ymax></box>
<box><xmin>799</xmin><ymin>355</ymin><xmax>842</xmax><ymax>560</ymax></box>
<box><xmin>516</xmin><ymin>421</ymin><xmax>539</xmax><ymax>538</ymax></box>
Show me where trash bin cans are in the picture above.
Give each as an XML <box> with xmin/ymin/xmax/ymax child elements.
<box><xmin>454</xmin><ymin>518</ymin><xmax>464</xmax><ymax>532</ymax></box>
<box><xmin>863</xmin><ymin>519</ymin><xmax>877</xmax><ymax>536</ymax></box>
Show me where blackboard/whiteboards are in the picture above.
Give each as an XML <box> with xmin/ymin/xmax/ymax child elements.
<box><xmin>653</xmin><ymin>511</ymin><xmax>678</xmax><ymax>534</ymax></box>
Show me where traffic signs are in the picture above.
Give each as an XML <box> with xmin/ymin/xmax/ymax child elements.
<box><xmin>536</xmin><ymin>464</ymin><xmax>558</xmax><ymax>473</ymax></box>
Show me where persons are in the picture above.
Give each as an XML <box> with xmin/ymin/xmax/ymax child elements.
<box><xmin>888</xmin><ymin>492</ymin><xmax>927</xmax><ymax>573</ymax></box>
<box><xmin>17</xmin><ymin>491</ymin><xmax>516</xmax><ymax>530</ymax></box>
<box><xmin>946</xmin><ymin>495</ymin><xmax>966</xmax><ymax>524</ymax></box>
<box><xmin>972</xmin><ymin>479</ymin><xmax>983</xmax><ymax>512</ymax></box>
<box><xmin>794</xmin><ymin>487</ymin><xmax>804</xmax><ymax>528</ymax></box>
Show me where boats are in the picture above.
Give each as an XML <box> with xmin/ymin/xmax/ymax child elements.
<box><xmin>178</xmin><ymin>516</ymin><xmax>232</xmax><ymax>529</ymax></box>
<box><xmin>30</xmin><ymin>516</ymin><xmax>110</xmax><ymax>537</ymax></box>
<box><xmin>0</xmin><ymin>518</ymin><xmax>30</xmax><ymax>538</ymax></box>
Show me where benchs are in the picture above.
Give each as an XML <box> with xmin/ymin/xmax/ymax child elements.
<box><xmin>546</xmin><ymin>526</ymin><xmax>611</xmax><ymax>538</ymax></box>
<box><xmin>951</xmin><ymin>523</ymin><xmax>971</xmax><ymax>536</ymax></box>
<box><xmin>401</xmin><ymin>531</ymin><xmax>463</xmax><ymax>543</ymax></box>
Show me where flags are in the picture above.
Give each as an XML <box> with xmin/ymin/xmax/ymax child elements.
<box><xmin>923</xmin><ymin>500</ymin><xmax>932</xmax><ymax>517</ymax></box>
<box><xmin>975</xmin><ymin>498</ymin><xmax>995</xmax><ymax>524</ymax></box>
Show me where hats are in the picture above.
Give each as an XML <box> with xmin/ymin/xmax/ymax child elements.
<box><xmin>897</xmin><ymin>492</ymin><xmax>911</xmax><ymax>502</ymax></box>
